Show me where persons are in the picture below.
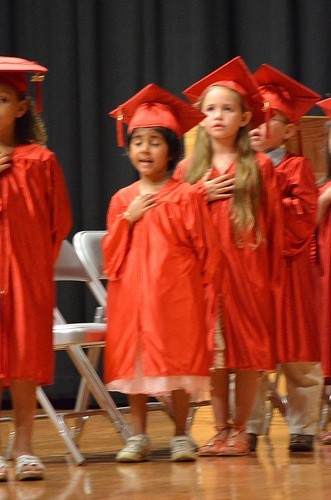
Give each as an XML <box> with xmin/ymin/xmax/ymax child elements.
<box><xmin>0</xmin><ymin>57</ymin><xmax>73</xmax><ymax>480</ymax></box>
<box><xmin>226</xmin><ymin>64</ymin><xmax>317</xmax><ymax>452</ymax></box>
<box><xmin>315</xmin><ymin>96</ymin><xmax>331</xmax><ymax>443</ymax></box>
<box><xmin>102</xmin><ymin>84</ymin><xmax>218</xmax><ymax>462</ymax></box>
<box><xmin>171</xmin><ymin>57</ymin><xmax>282</xmax><ymax>456</ymax></box>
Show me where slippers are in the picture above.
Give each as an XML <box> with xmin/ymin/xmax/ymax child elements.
<box><xmin>14</xmin><ymin>454</ymin><xmax>45</xmax><ymax>480</ymax></box>
<box><xmin>0</xmin><ymin>456</ymin><xmax>9</xmax><ymax>481</ymax></box>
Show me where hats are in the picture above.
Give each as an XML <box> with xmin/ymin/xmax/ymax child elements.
<box><xmin>108</xmin><ymin>84</ymin><xmax>207</xmax><ymax>147</ymax></box>
<box><xmin>182</xmin><ymin>56</ymin><xmax>270</xmax><ymax>140</ymax></box>
<box><xmin>316</xmin><ymin>97</ymin><xmax>331</xmax><ymax>118</ymax></box>
<box><xmin>253</xmin><ymin>63</ymin><xmax>322</xmax><ymax>156</ymax></box>
<box><xmin>0</xmin><ymin>56</ymin><xmax>48</xmax><ymax>112</ymax></box>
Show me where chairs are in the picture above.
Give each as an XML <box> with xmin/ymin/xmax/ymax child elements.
<box><xmin>5</xmin><ymin>230</ymin><xmax>287</xmax><ymax>465</ymax></box>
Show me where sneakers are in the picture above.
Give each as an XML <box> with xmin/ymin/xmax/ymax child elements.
<box><xmin>170</xmin><ymin>434</ymin><xmax>197</xmax><ymax>461</ymax></box>
<box><xmin>116</xmin><ymin>436</ymin><xmax>151</xmax><ymax>461</ymax></box>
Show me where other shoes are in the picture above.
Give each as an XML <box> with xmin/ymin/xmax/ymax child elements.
<box><xmin>248</xmin><ymin>433</ymin><xmax>257</xmax><ymax>451</ymax></box>
<box><xmin>320</xmin><ymin>429</ymin><xmax>331</xmax><ymax>442</ymax></box>
<box><xmin>289</xmin><ymin>435</ymin><xmax>314</xmax><ymax>451</ymax></box>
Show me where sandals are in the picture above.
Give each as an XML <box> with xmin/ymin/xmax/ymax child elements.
<box><xmin>198</xmin><ymin>426</ymin><xmax>229</xmax><ymax>455</ymax></box>
<box><xmin>218</xmin><ymin>425</ymin><xmax>250</xmax><ymax>456</ymax></box>
